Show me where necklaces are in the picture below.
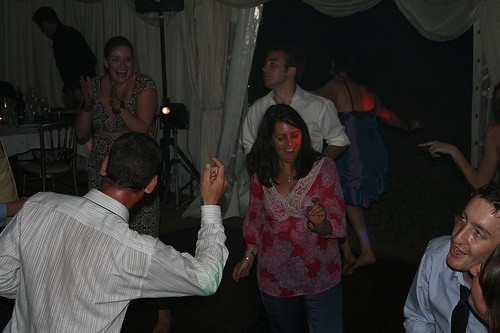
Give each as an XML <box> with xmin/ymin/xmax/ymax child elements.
<box><xmin>279</xmin><ymin>173</ymin><xmax>296</xmax><ymax>184</ymax></box>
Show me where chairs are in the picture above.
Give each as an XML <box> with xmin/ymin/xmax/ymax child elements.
<box><xmin>18</xmin><ymin>117</ymin><xmax>78</xmax><ymax>198</ymax></box>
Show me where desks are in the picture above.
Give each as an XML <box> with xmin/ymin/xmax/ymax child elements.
<box><xmin>1</xmin><ymin>124</ymin><xmax>91</xmax><ymax>170</ymax></box>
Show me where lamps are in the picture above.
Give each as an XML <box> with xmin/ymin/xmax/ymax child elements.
<box><xmin>159</xmin><ymin>103</ymin><xmax>190</xmax><ymax>130</ymax></box>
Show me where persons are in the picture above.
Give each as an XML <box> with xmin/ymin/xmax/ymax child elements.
<box><xmin>33</xmin><ymin>6</ymin><xmax>97</xmax><ymax>108</ymax></box>
<box><xmin>0</xmin><ymin>36</ymin><xmax>500</xmax><ymax>333</ymax></box>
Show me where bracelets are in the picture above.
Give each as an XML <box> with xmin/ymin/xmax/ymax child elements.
<box><xmin>81</xmin><ymin>103</ymin><xmax>92</xmax><ymax>112</ymax></box>
<box><xmin>111</xmin><ymin>100</ymin><xmax>125</xmax><ymax>115</ymax></box>
<box><xmin>405</xmin><ymin>121</ymin><xmax>411</xmax><ymax>130</ymax></box>
<box><xmin>307</xmin><ymin>220</ymin><xmax>328</xmax><ymax>234</ymax></box>
<box><xmin>245</xmin><ymin>248</ymin><xmax>255</xmax><ymax>262</ymax></box>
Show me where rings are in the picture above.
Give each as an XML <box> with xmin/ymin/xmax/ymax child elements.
<box><xmin>415</xmin><ymin>123</ymin><xmax>418</xmax><ymax>126</ymax></box>
<box><xmin>431</xmin><ymin>147</ymin><xmax>433</xmax><ymax>151</ymax></box>
